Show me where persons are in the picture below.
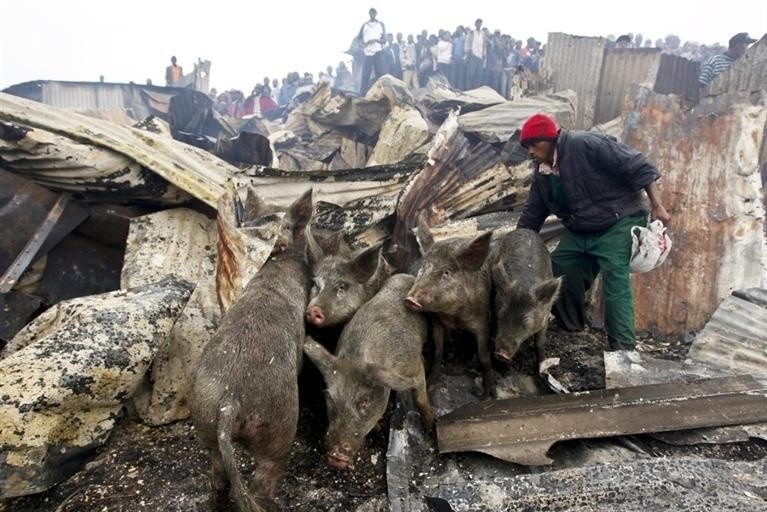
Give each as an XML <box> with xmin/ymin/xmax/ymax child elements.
<box><xmin>514</xmin><ymin>113</ymin><xmax>672</xmax><ymax>350</ymax></box>
<box><xmin>608</xmin><ymin>31</ymin><xmax>759</xmax><ymax>85</ymax></box>
<box><xmin>166</xmin><ymin>57</ymin><xmax>182</xmax><ymax>86</ymax></box>
<box><xmin>212</xmin><ymin>8</ymin><xmax>544</xmax><ymax>119</ymax></box>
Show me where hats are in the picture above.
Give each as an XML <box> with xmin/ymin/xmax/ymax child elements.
<box><xmin>729</xmin><ymin>32</ymin><xmax>758</xmax><ymax>48</ymax></box>
<box><xmin>520</xmin><ymin>114</ymin><xmax>558</xmax><ymax>149</ymax></box>
<box><xmin>616</xmin><ymin>35</ymin><xmax>630</xmax><ymax>43</ymax></box>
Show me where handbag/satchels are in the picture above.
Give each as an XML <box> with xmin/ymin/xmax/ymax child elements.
<box><xmin>631</xmin><ymin>221</ymin><xmax>674</xmax><ymax>277</ymax></box>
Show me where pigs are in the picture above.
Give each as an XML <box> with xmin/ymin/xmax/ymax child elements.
<box><xmin>302</xmin><ymin>223</ymin><xmax>392</xmax><ymax>327</ymax></box>
<box><xmin>302</xmin><ymin>272</ymin><xmax>433</xmax><ymax>467</ymax></box>
<box><xmin>488</xmin><ymin>227</ymin><xmax>565</xmax><ymax>378</ymax></box>
<box><xmin>187</xmin><ymin>185</ymin><xmax>345</xmax><ymax>512</ymax></box>
<box><xmin>403</xmin><ymin>209</ymin><xmax>496</xmax><ymax>390</ymax></box>
